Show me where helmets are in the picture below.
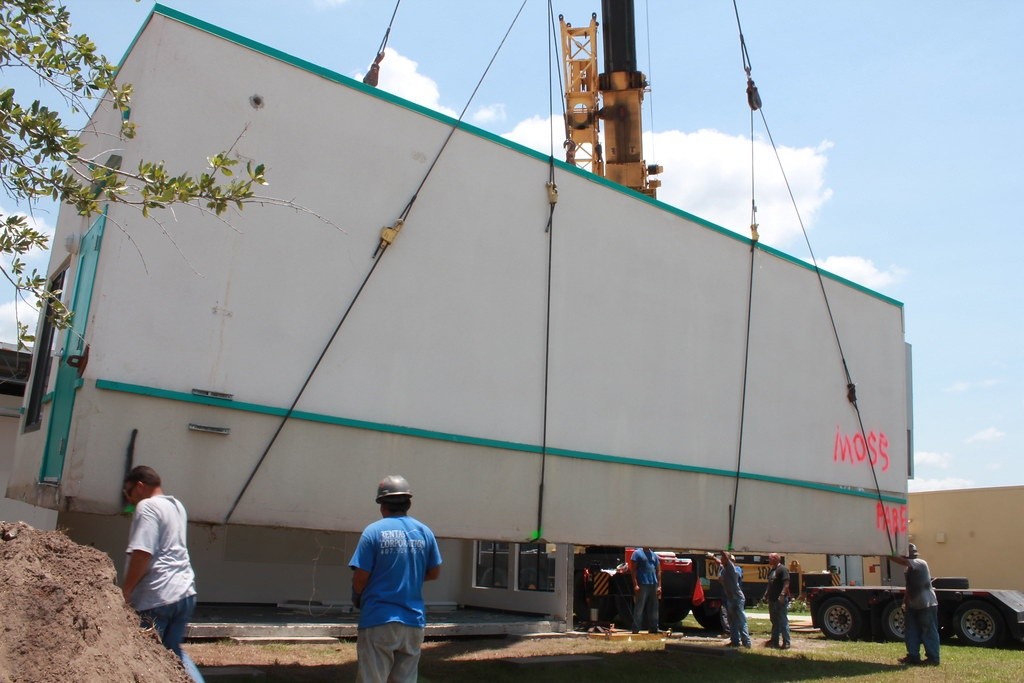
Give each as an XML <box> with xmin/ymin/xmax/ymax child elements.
<box><xmin>375</xmin><ymin>475</ymin><xmax>413</xmax><ymax>504</ymax></box>
<box><xmin>617</xmin><ymin>562</ymin><xmax>628</xmax><ymax>573</ymax></box>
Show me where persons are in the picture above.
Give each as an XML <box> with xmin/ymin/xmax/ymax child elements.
<box><xmin>891</xmin><ymin>543</ymin><xmax>940</xmax><ymax>666</ymax></box>
<box><xmin>762</xmin><ymin>553</ymin><xmax>791</xmax><ymax>649</ymax></box>
<box><xmin>706</xmin><ymin>551</ymin><xmax>751</xmax><ymax>649</ymax></box>
<box><xmin>122</xmin><ymin>466</ymin><xmax>205</xmax><ymax>683</ymax></box>
<box><xmin>347</xmin><ymin>476</ymin><xmax>441</xmax><ymax>683</ymax></box>
<box><xmin>630</xmin><ymin>547</ymin><xmax>662</xmax><ymax>634</ymax></box>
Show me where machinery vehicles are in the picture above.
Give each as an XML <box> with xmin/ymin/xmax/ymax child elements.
<box><xmin>478</xmin><ymin>1</ymin><xmax>786</xmax><ymax>635</ymax></box>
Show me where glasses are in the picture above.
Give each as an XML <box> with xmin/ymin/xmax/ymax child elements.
<box><xmin>126</xmin><ymin>482</ymin><xmax>139</xmax><ymax>498</ymax></box>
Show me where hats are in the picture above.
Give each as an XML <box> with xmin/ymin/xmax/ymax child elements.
<box><xmin>730</xmin><ymin>554</ymin><xmax>735</xmax><ymax>560</ymax></box>
<box><xmin>908</xmin><ymin>543</ymin><xmax>918</xmax><ymax>556</ymax></box>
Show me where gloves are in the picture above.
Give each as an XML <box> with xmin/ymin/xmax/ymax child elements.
<box><xmin>705</xmin><ymin>552</ymin><xmax>714</xmax><ymax>558</ymax></box>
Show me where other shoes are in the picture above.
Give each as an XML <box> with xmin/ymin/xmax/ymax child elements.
<box><xmin>726</xmin><ymin>642</ymin><xmax>741</xmax><ymax>647</ymax></box>
<box><xmin>898</xmin><ymin>655</ymin><xmax>921</xmax><ymax>664</ymax></box>
<box><xmin>781</xmin><ymin>641</ymin><xmax>790</xmax><ymax>649</ymax></box>
<box><xmin>649</xmin><ymin>629</ymin><xmax>663</xmax><ymax>633</ymax></box>
<box><xmin>921</xmin><ymin>658</ymin><xmax>940</xmax><ymax>666</ymax></box>
<box><xmin>764</xmin><ymin>640</ymin><xmax>780</xmax><ymax>648</ymax></box>
